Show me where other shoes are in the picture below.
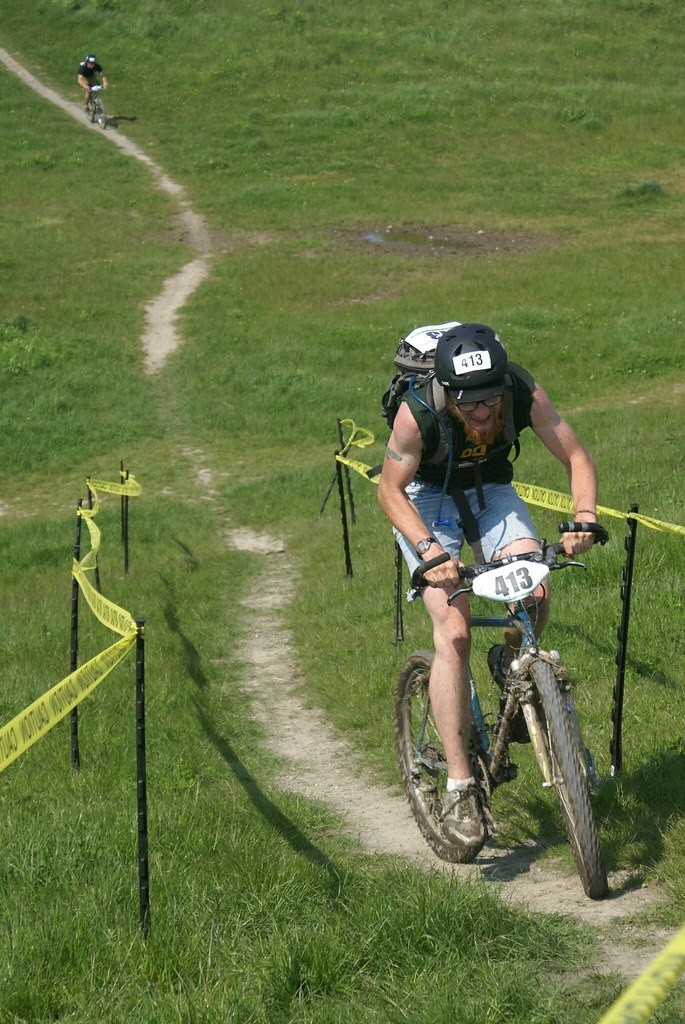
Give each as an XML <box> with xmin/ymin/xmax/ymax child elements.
<box><xmin>83</xmin><ymin>106</ymin><xmax>89</xmax><ymax>112</ymax></box>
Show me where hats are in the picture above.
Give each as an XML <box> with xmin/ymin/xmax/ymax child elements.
<box><xmin>447</xmin><ymin>374</ymin><xmax>506</xmax><ymax>403</ymax></box>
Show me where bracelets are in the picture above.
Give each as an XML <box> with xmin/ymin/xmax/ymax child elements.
<box><xmin>576</xmin><ymin>510</ymin><xmax>596</xmax><ymax>516</ymax></box>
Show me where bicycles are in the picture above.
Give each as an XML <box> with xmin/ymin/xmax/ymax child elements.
<box><xmin>389</xmin><ymin>519</ymin><xmax>611</xmax><ymax>902</ymax></box>
<box><xmin>84</xmin><ymin>82</ymin><xmax>109</xmax><ymax>129</ymax></box>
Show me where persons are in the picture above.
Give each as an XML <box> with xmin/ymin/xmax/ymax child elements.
<box><xmin>377</xmin><ymin>323</ymin><xmax>597</xmax><ymax>849</ymax></box>
<box><xmin>78</xmin><ymin>54</ymin><xmax>108</xmax><ymax>109</ymax></box>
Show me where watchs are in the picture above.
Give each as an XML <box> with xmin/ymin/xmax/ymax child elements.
<box><xmin>417</xmin><ymin>537</ymin><xmax>436</xmax><ymax>560</ymax></box>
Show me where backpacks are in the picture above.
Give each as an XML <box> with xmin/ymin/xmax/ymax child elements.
<box><xmin>381</xmin><ymin>319</ymin><xmax>519</xmax><ymax>464</ymax></box>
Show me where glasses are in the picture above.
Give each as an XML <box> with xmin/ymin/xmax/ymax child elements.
<box><xmin>452</xmin><ymin>393</ymin><xmax>504</xmax><ymax>412</ymax></box>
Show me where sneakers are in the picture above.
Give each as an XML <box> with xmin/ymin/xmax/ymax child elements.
<box><xmin>488</xmin><ymin>643</ymin><xmax>532</xmax><ymax>745</ymax></box>
<box><xmin>439</xmin><ymin>783</ymin><xmax>490</xmax><ymax>845</ymax></box>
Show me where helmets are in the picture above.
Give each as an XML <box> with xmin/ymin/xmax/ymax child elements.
<box><xmin>434</xmin><ymin>323</ymin><xmax>508</xmax><ymax>389</ymax></box>
<box><xmin>85</xmin><ymin>54</ymin><xmax>96</xmax><ymax>63</ymax></box>
